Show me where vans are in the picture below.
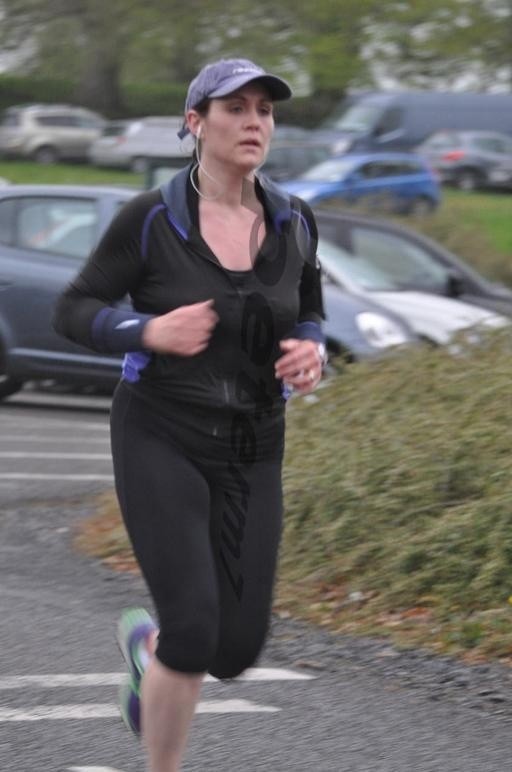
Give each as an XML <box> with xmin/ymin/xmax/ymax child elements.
<box><xmin>294</xmin><ymin>88</ymin><xmax>511</xmax><ymax>168</ymax></box>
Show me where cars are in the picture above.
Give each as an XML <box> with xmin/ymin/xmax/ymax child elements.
<box><xmin>2</xmin><ymin>185</ymin><xmax>511</xmax><ymax>398</ymax></box>
<box><xmin>309</xmin><ymin>210</ymin><xmax>512</xmax><ymax>321</ymax></box>
<box><xmin>271</xmin><ymin>151</ymin><xmax>442</xmax><ymax>220</ymax></box>
<box><xmin>411</xmin><ymin>129</ymin><xmax>512</xmax><ymax>194</ymax></box>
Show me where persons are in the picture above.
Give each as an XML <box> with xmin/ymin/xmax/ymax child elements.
<box><xmin>52</xmin><ymin>57</ymin><xmax>326</xmax><ymax>772</ymax></box>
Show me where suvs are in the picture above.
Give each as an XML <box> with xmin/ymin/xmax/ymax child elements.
<box><xmin>1</xmin><ymin>101</ymin><xmax>110</xmax><ymax>168</ymax></box>
<box><xmin>83</xmin><ymin>114</ymin><xmax>199</xmax><ymax>173</ymax></box>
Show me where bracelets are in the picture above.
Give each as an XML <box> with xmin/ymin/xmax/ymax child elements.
<box><xmin>314</xmin><ymin>341</ymin><xmax>328</xmax><ymax>365</ymax></box>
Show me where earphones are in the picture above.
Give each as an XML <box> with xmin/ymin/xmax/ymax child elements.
<box><xmin>196</xmin><ymin>123</ymin><xmax>202</xmax><ymax>140</ymax></box>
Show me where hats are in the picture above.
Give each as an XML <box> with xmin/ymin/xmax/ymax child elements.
<box><xmin>184</xmin><ymin>58</ymin><xmax>292</xmax><ymax>119</ymax></box>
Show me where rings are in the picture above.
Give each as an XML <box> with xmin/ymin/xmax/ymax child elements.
<box><xmin>307</xmin><ymin>369</ymin><xmax>317</xmax><ymax>381</ymax></box>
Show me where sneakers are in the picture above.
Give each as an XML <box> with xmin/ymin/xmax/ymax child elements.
<box><xmin>116</xmin><ymin>607</ymin><xmax>167</xmax><ymax>741</ymax></box>
